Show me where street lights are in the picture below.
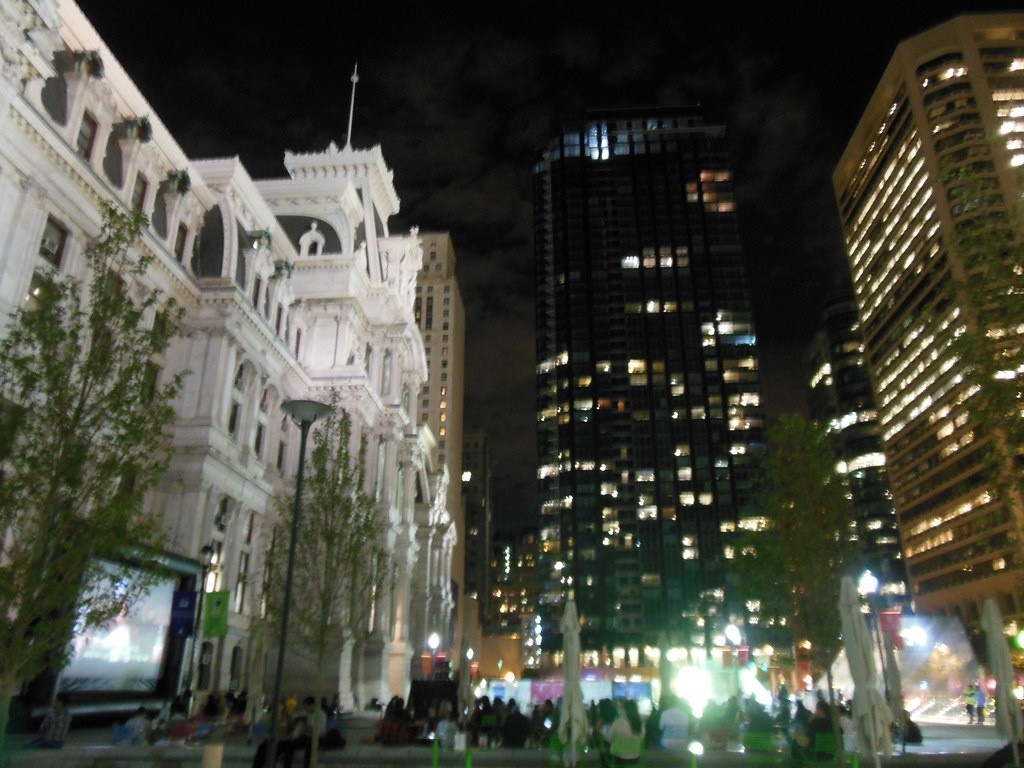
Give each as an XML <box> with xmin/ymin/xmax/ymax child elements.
<box><xmin>858</xmin><ymin>572</ymin><xmax>891</xmax><ymax>708</ymax></box>
<box><xmin>267</xmin><ymin>398</ymin><xmax>334</xmax><ymax>743</ymax></box>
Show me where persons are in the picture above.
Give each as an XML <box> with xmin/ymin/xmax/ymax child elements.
<box><xmin>964</xmin><ymin>684</ymin><xmax>986</xmax><ymax>725</ymax></box>
<box><xmin>22</xmin><ymin>692</ymin><xmax>72</xmax><ymax>748</ymax></box>
<box><xmin>124</xmin><ymin>687</ymin><xmax>340</xmax><ymax>768</ymax></box>
<box><xmin>383</xmin><ymin>684</ymin><xmax>923</xmax><ymax>768</ymax></box>
